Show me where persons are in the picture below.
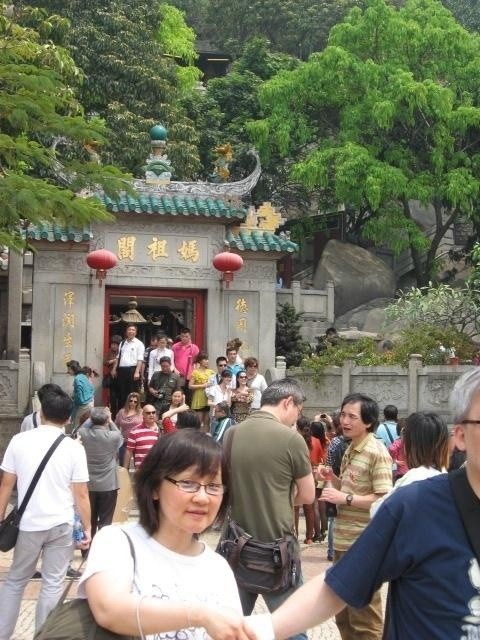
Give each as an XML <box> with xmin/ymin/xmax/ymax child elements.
<box><xmin>0</xmin><ymin>323</ymin><xmax>480</xmax><ymax>639</ymax></box>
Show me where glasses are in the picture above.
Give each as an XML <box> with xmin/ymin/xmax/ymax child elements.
<box><xmin>145</xmin><ymin>411</ymin><xmax>155</xmax><ymax>415</ymax></box>
<box><xmin>166</xmin><ymin>477</ymin><xmax>226</xmax><ymax>496</ymax></box>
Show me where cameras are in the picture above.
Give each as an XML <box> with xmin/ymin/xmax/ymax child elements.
<box><xmin>156</xmin><ymin>390</ymin><xmax>166</xmax><ymax>398</ymax></box>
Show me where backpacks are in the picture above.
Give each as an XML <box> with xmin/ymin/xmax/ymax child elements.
<box><xmin>333</xmin><ymin>435</ymin><xmax>350</xmax><ymax>475</ymax></box>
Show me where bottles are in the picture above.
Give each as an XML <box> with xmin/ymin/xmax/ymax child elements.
<box><xmin>72</xmin><ymin>513</ymin><xmax>84</xmax><ymax>552</ymax></box>
<box><xmin>323</xmin><ymin>475</ymin><xmax>338</xmax><ymax>518</ymax></box>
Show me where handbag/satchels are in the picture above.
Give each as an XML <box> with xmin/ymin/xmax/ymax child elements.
<box><xmin>218</xmin><ymin>518</ymin><xmax>297</xmax><ymax>596</ymax></box>
<box><xmin>34</xmin><ymin>598</ymin><xmax>124</xmax><ymax>640</ymax></box>
<box><xmin>1</xmin><ymin>510</ymin><xmax>20</xmax><ymax>551</ymax></box>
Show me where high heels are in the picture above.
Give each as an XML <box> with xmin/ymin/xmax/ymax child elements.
<box><xmin>304</xmin><ymin>533</ymin><xmax>326</xmax><ymax>545</ymax></box>
